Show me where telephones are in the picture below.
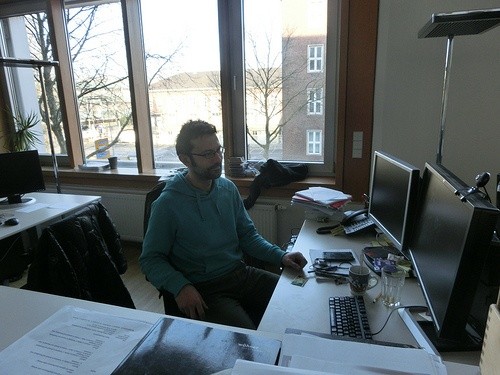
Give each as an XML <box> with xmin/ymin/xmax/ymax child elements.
<box><xmin>340</xmin><ymin>209</ymin><xmax>375</xmax><ymax>235</ymax></box>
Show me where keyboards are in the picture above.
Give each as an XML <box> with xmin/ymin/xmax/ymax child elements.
<box><xmin>330</xmin><ymin>295</ymin><xmax>373</xmax><ymax>340</ymax></box>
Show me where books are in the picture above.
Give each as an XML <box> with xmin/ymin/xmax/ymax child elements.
<box><xmin>292</xmin><ymin>187</ymin><xmax>353</xmax><ymax>219</ymax></box>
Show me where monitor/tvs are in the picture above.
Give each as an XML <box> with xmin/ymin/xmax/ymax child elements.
<box><xmin>363</xmin><ymin>150</ymin><xmax>422</xmax><ymax>266</ymax></box>
<box><xmin>399</xmin><ymin>164</ymin><xmax>491</xmax><ymax>355</ymax></box>
<box><xmin>0</xmin><ymin>149</ymin><xmax>46</xmax><ymax>205</ymax></box>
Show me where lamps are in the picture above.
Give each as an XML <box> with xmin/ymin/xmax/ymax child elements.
<box><xmin>418</xmin><ymin>11</ymin><xmax>500</xmax><ymax>165</ymax></box>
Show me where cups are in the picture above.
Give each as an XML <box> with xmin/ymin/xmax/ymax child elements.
<box><xmin>381</xmin><ymin>264</ymin><xmax>408</xmax><ymax>308</ymax></box>
<box><xmin>108</xmin><ymin>157</ymin><xmax>118</xmax><ymax>170</ymax></box>
<box><xmin>348</xmin><ymin>265</ymin><xmax>379</xmax><ymax>297</ymax></box>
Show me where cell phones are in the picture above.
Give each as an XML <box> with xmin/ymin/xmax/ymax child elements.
<box><xmin>322</xmin><ymin>251</ymin><xmax>356</xmax><ymax>261</ymax></box>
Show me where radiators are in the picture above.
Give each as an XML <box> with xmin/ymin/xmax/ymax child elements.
<box><xmin>43</xmin><ymin>189</ymin><xmax>278</xmax><ymax>245</ymax></box>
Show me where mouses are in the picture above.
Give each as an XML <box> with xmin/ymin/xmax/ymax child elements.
<box><xmin>5</xmin><ymin>218</ymin><xmax>19</xmax><ymax>225</ymax></box>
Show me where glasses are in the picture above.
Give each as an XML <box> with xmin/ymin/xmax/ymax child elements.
<box><xmin>188</xmin><ymin>146</ymin><xmax>224</xmax><ymax>158</ymax></box>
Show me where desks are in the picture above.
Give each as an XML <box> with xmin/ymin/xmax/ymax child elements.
<box><xmin>258</xmin><ymin>219</ymin><xmax>482</xmax><ymax>366</ymax></box>
<box><xmin>0</xmin><ymin>193</ymin><xmax>102</xmax><ymax>239</ymax></box>
<box><xmin>0</xmin><ymin>285</ymin><xmax>481</xmax><ymax>375</ymax></box>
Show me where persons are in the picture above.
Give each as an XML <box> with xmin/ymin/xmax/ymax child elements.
<box><xmin>138</xmin><ymin>120</ymin><xmax>308</xmax><ymax>329</ymax></box>
